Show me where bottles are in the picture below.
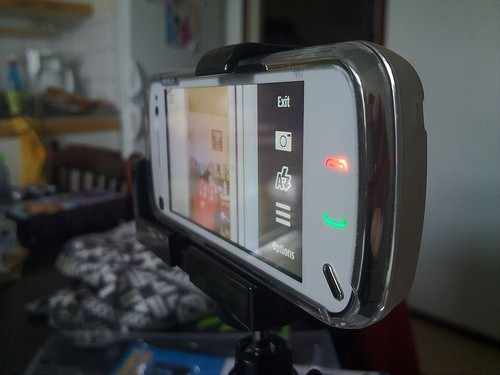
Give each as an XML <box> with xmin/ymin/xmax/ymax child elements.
<box><xmin>6</xmin><ymin>59</ymin><xmax>24</xmax><ymax>116</ymax></box>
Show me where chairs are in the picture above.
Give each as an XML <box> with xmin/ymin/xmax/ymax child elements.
<box><xmin>44</xmin><ymin>140</ymin><xmax>141</xmax><ymax>197</ymax></box>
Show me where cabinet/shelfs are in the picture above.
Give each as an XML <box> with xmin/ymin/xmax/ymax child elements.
<box><xmin>0</xmin><ymin>115</ymin><xmax>120</xmax><ymax>187</ymax></box>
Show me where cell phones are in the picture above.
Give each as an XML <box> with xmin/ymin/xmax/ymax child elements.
<box><xmin>144</xmin><ymin>41</ymin><xmax>428</xmax><ymax>330</ymax></box>
